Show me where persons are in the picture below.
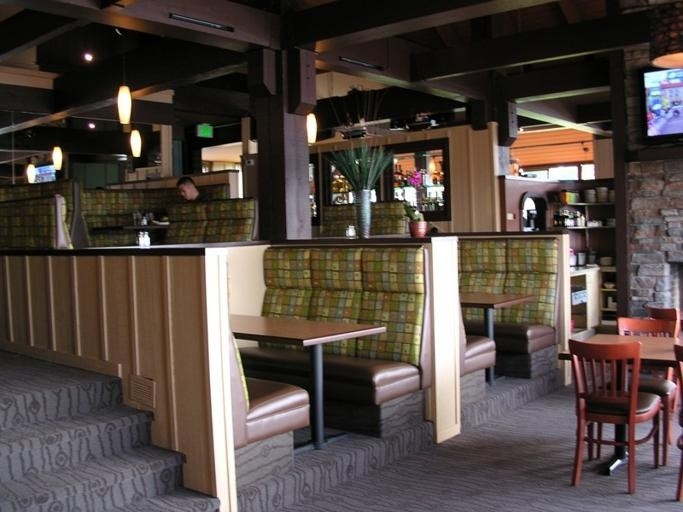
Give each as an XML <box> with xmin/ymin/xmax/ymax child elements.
<box><xmin>175</xmin><ymin>177</ymin><xmax>203</xmax><ymax>201</ymax></box>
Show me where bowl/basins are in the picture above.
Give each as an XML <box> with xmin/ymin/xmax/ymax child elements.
<box><xmin>599</xmin><ymin>256</ymin><xmax>613</xmax><ymax>267</ymax></box>
<box><xmin>603</xmin><ymin>283</ymin><xmax>616</xmax><ymax>289</ymax></box>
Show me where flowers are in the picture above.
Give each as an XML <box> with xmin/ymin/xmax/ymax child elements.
<box><xmin>401</xmin><ymin>168</ymin><xmax>427</xmax><ymax>188</ymax></box>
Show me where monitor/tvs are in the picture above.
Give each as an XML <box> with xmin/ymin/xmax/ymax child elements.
<box><xmin>26</xmin><ymin>162</ymin><xmax>68</xmax><ymax>184</ymax></box>
<box><xmin>637</xmin><ymin>62</ymin><xmax>683</xmax><ymax>148</ymax></box>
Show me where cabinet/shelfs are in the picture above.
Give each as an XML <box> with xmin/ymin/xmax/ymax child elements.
<box><xmin>558</xmin><ymin>179</ymin><xmax>626</xmax><ymax>326</ymax></box>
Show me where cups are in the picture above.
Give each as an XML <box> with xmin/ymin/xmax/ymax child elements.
<box><xmin>584</xmin><ymin>187</ymin><xmax>614</xmax><ymax>202</ymax></box>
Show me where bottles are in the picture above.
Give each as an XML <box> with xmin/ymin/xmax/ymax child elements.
<box><xmin>135</xmin><ymin>230</ymin><xmax>151</xmax><ymax>246</ymax></box>
<box><xmin>553</xmin><ymin>210</ymin><xmax>585</xmax><ymax>228</ymax></box>
<box><xmin>346</xmin><ymin>224</ymin><xmax>355</xmax><ymax>238</ymax></box>
<box><xmin>140</xmin><ymin>216</ymin><xmax>148</xmax><ymax>225</ymax></box>
<box><xmin>569</xmin><ymin>249</ymin><xmax>594</xmax><ymax>266</ymax></box>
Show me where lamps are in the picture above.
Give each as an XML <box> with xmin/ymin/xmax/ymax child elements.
<box><xmin>650</xmin><ymin>2</ymin><xmax>681</xmax><ymax>69</ymax></box>
<box><xmin>116</xmin><ymin>31</ymin><xmax>142</xmax><ymax>158</ymax></box>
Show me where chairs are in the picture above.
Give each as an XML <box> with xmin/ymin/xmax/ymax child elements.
<box><xmin>557</xmin><ymin>306</ymin><xmax>683</xmax><ymax>501</ymax></box>
<box><xmin>0</xmin><ymin>179</ymin><xmax>258</xmax><ymax>249</ymax></box>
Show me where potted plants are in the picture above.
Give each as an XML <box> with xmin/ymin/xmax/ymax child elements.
<box><xmin>323</xmin><ymin>134</ymin><xmax>395</xmax><ymax>236</ymax></box>
<box><xmin>403</xmin><ymin>204</ymin><xmax>428</xmax><ymax>239</ymax></box>
